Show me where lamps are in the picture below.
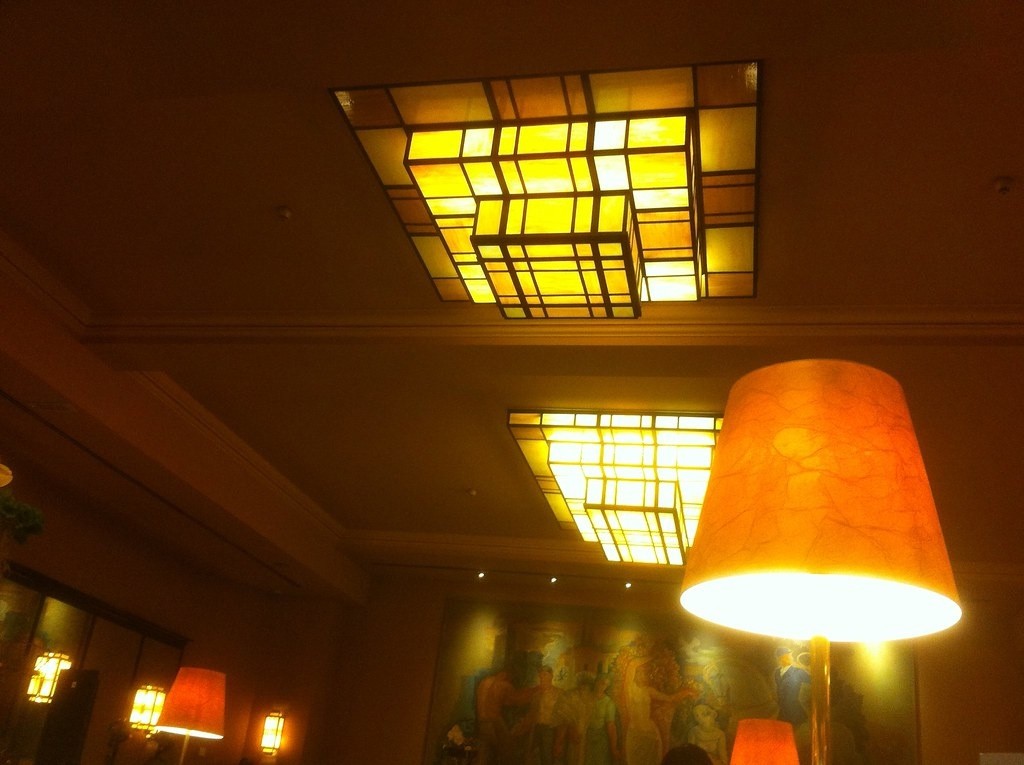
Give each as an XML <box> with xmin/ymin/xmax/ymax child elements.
<box><xmin>679</xmin><ymin>359</ymin><xmax>964</xmax><ymax>765</ymax></box>
<box><xmin>729</xmin><ymin>718</ymin><xmax>800</xmax><ymax>765</ymax></box>
<box><xmin>261</xmin><ymin>709</ymin><xmax>285</xmax><ymax>756</ymax></box>
<box><xmin>26</xmin><ymin>651</ymin><xmax>50</xmax><ymax>702</ymax></box>
<box><xmin>154</xmin><ymin>666</ymin><xmax>228</xmax><ymax>765</ymax></box>
<box><xmin>129</xmin><ymin>684</ymin><xmax>147</xmax><ymax>729</ymax></box>
<box><xmin>506</xmin><ymin>412</ymin><xmax>724</xmax><ymax>567</ymax></box>
<box><xmin>139</xmin><ymin>686</ymin><xmax>166</xmax><ymax>732</ymax></box>
<box><xmin>401</xmin><ymin>111</ymin><xmax>703</xmax><ymax>319</ymax></box>
<box><xmin>37</xmin><ymin>652</ymin><xmax>72</xmax><ymax>704</ymax></box>
